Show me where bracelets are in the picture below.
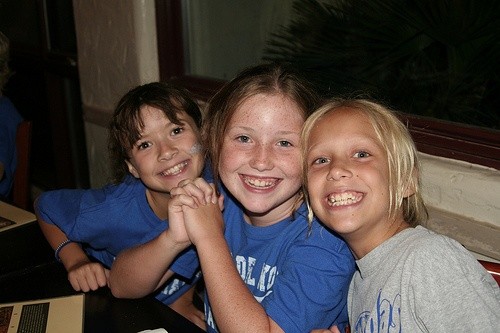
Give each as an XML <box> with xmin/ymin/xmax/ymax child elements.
<box><xmin>55</xmin><ymin>239</ymin><xmax>82</xmax><ymax>263</ymax></box>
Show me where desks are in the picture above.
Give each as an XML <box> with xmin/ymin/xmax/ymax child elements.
<box><xmin>0</xmin><ymin>199</ymin><xmax>207</xmax><ymax>333</ymax></box>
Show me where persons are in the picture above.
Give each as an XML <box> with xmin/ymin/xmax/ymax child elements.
<box><xmin>107</xmin><ymin>61</ymin><xmax>356</xmax><ymax>333</ymax></box>
<box><xmin>0</xmin><ymin>33</ymin><xmax>29</xmax><ymax>206</ymax></box>
<box><xmin>28</xmin><ymin>82</ymin><xmax>226</xmax><ymax>331</ymax></box>
<box><xmin>302</xmin><ymin>98</ymin><xmax>500</xmax><ymax>333</ymax></box>
